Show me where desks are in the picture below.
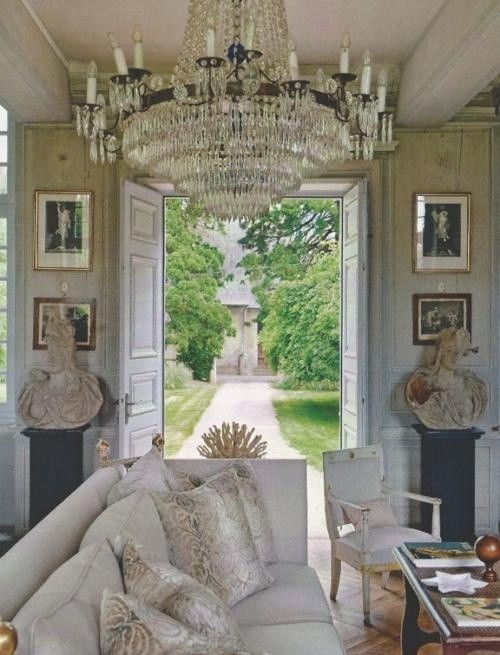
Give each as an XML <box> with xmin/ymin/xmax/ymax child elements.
<box><xmin>410</xmin><ymin>422</ymin><xmax>486</xmax><ymax>545</ymax></box>
<box><xmin>20</xmin><ymin>423</ymin><xmax>93</xmax><ymax>532</ymax></box>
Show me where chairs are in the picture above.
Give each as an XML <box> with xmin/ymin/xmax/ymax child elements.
<box><xmin>321</xmin><ymin>447</ymin><xmax>442</xmax><ymax>626</ymax></box>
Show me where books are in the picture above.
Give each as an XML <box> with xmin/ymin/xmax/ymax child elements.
<box><xmin>401</xmin><ymin>541</ymin><xmax>485</xmax><ymax>568</ymax></box>
<box><xmin>440</xmin><ymin>597</ymin><xmax>500</xmax><ymax>627</ymax></box>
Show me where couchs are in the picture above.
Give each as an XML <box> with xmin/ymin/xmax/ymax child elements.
<box><xmin>0</xmin><ymin>439</ymin><xmax>347</xmax><ymax>655</ymax></box>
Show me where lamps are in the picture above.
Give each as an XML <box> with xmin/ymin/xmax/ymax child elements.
<box><xmin>71</xmin><ymin>0</ymin><xmax>401</xmax><ymax>227</ymax></box>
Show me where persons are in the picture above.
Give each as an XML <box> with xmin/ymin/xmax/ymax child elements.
<box><xmin>405</xmin><ymin>327</ymin><xmax>485</xmax><ymax>430</ymax></box>
<box><xmin>16</xmin><ymin>317</ymin><xmax>103</xmax><ymax>430</ymax></box>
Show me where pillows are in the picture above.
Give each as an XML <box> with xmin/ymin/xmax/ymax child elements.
<box><xmin>16</xmin><ymin>536</ymin><xmax>125</xmax><ymax>654</ymax></box>
<box><xmin>106</xmin><ymin>452</ymin><xmax>168</xmax><ymax>508</ymax></box>
<box><xmin>78</xmin><ymin>485</ymin><xmax>167</xmax><ymax>562</ymax></box>
<box><xmin>100</xmin><ymin>587</ymin><xmax>254</xmax><ymax>654</ymax></box>
<box><xmin>147</xmin><ymin>466</ymin><xmax>274</xmax><ymax>608</ymax></box>
<box><xmin>120</xmin><ymin>537</ymin><xmax>251</xmax><ymax>651</ymax></box>
<box><xmin>166</xmin><ymin>457</ymin><xmax>279</xmax><ymax>566</ymax></box>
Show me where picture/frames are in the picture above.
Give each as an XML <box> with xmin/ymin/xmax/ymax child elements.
<box><xmin>409</xmin><ymin>190</ymin><xmax>472</xmax><ymax>274</ymax></box>
<box><xmin>31</xmin><ymin>188</ymin><xmax>96</xmax><ymax>273</ymax></box>
<box><xmin>411</xmin><ymin>291</ymin><xmax>472</xmax><ymax>348</ymax></box>
<box><xmin>32</xmin><ymin>296</ymin><xmax>97</xmax><ymax>352</ymax></box>
